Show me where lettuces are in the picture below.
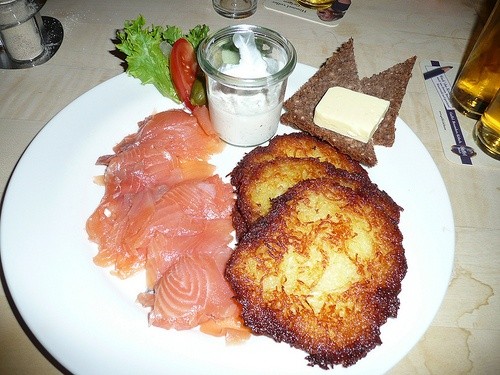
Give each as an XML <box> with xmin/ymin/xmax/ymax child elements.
<box><xmin>113</xmin><ymin>14</ymin><xmax>211</xmax><ymax>104</ymax></box>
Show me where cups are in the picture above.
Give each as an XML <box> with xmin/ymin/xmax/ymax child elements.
<box><xmin>212</xmin><ymin>0</ymin><xmax>258</xmax><ymax>19</ymax></box>
<box><xmin>450</xmin><ymin>0</ymin><xmax>500</xmax><ymax>160</ymax></box>
<box><xmin>296</xmin><ymin>0</ymin><xmax>336</xmax><ymax>10</ymax></box>
<box><xmin>196</xmin><ymin>23</ymin><xmax>297</xmax><ymax>146</ymax></box>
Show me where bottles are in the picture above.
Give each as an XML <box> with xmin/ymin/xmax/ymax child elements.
<box><xmin>0</xmin><ymin>0</ymin><xmax>45</xmax><ymax>60</ymax></box>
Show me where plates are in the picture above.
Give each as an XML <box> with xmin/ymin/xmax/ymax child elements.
<box><xmin>0</xmin><ymin>62</ymin><xmax>454</xmax><ymax>371</ymax></box>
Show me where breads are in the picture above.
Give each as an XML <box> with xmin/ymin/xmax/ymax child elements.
<box><xmin>280</xmin><ymin>36</ymin><xmax>417</xmax><ymax>168</ymax></box>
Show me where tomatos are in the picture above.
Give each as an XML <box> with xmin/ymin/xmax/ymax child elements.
<box><xmin>170</xmin><ymin>38</ymin><xmax>200</xmax><ymax>112</ymax></box>
